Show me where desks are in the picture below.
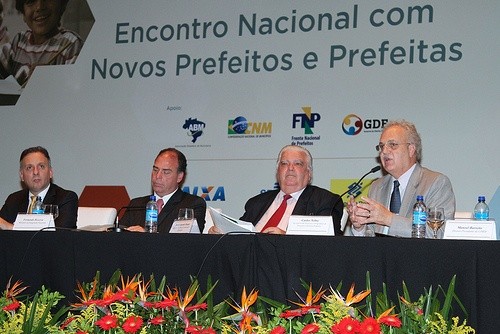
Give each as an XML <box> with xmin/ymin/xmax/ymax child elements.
<box><xmin>0</xmin><ymin>229</ymin><xmax>500</xmax><ymax>334</ymax></box>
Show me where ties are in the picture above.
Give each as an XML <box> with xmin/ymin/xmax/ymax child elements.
<box><xmin>259</xmin><ymin>194</ymin><xmax>292</xmax><ymax>233</ymax></box>
<box><xmin>156</xmin><ymin>199</ymin><xmax>164</xmax><ymax>215</ymax></box>
<box><xmin>389</xmin><ymin>180</ymin><xmax>401</xmax><ymax>213</ymax></box>
<box><xmin>28</xmin><ymin>196</ymin><xmax>37</xmax><ymax>214</ymax></box>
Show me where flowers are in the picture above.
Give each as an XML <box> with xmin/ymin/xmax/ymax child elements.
<box><xmin>0</xmin><ymin>276</ymin><xmax>475</xmax><ymax>334</ymax></box>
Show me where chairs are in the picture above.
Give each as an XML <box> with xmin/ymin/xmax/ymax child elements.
<box><xmin>76</xmin><ymin>207</ymin><xmax>119</xmax><ymax>230</ymax></box>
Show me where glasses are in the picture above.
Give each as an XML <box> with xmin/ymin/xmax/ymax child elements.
<box><xmin>375</xmin><ymin>143</ymin><xmax>411</xmax><ymax>152</ymax></box>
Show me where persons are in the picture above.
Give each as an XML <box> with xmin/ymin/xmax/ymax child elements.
<box><xmin>119</xmin><ymin>148</ymin><xmax>207</xmax><ymax>234</ymax></box>
<box><xmin>0</xmin><ymin>0</ymin><xmax>84</xmax><ymax>89</ymax></box>
<box><xmin>343</xmin><ymin>121</ymin><xmax>456</xmax><ymax>239</ymax></box>
<box><xmin>0</xmin><ymin>146</ymin><xmax>78</xmax><ymax>230</ymax></box>
<box><xmin>208</xmin><ymin>145</ymin><xmax>344</xmax><ymax>235</ymax></box>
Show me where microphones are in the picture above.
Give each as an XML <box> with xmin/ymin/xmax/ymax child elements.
<box><xmin>329</xmin><ymin>166</ymin><xmax>380</xmax><ymax>214</ymax></box>
